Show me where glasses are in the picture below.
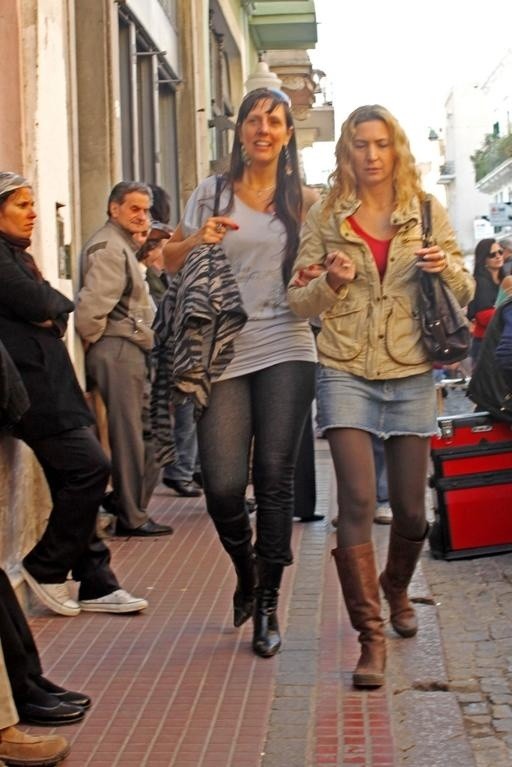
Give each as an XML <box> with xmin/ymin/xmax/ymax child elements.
<box><xmin>486</xmin><ymin>248</ymin><xmax>504</xmax><ymax>259</ymax></box>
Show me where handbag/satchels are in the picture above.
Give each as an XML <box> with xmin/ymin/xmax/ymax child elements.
<box><xmin>413</xmin><ymin>194</ymin><xmax>472</xmax><ymax>365</ymax></box>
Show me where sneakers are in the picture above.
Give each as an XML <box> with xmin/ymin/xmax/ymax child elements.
<box><xmin>79</xmin><ymin>590</ymin><xmax>154</xmax><ymax>620</ymax></box>
<box><xmin>0</xmin><ymin>725</ymin><xmax>72</xmax><ymax>767</ymax></box>
<box><xmin>17</xmin><ymin>556</ymin><xmax>82</xmax><ymax>620</ymax></box>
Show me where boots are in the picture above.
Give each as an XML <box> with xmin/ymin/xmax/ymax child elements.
<box><xmin>377</xmin><ymin>515</ymin><xmax>432</xmax><ymax>646</ymax></box>
<box><xmin>250</xmin><ymin>556</ymin><xmax>285</xmax><ymax>657</ymax></box>
<box><xmin>325</xmin><ymin>537</ymin><xmax>389</xmax><ymax>689</ymax></box>
<box><xmin>226</xmin><ymin>544</ymin><xmax>259</xmax><ymax>631</ymax></box>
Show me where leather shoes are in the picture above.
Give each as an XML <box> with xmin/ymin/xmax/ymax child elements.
<box><xmin>16</xmin><ymin>697</ymin><xmax>89</xmax><ymax>723</ymax></box>
<box><xmin>47</xmin><ymin>685</ymin><xmax>100</xmax><ymax>707</ymax></box>
<box><xmin>100</xmin><ymin>473</ymin><xmax>202</xmax><ymax>538</ymax></box>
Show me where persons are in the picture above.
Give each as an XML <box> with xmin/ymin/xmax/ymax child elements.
<box><xmin>162</xmin><ymin>88</ymin><xmax>327</xmax><ymax>659</ymax></box>
<box><xmin>0</xmin><ymin>631</ymin><xmax>72</xmax><ymax>767</ymax></box>
<box><xmin>0</xmin><ymin>560</ymin><xmax>92</xmax><ymax>728</ymax></box>
<box><xmin>75</xmin><ymin>180</ymin><xmax>172</xmax><ymax>537</ymax></box>
<box><xmin>287</xmin><ymin>104</ymin><xmax>477</xmax><ymax>690</ymax></box>
<box><xmin>1</xmin><ymin>170</ymin><xmax>148</xmax><ymax>616</ymax></box>
